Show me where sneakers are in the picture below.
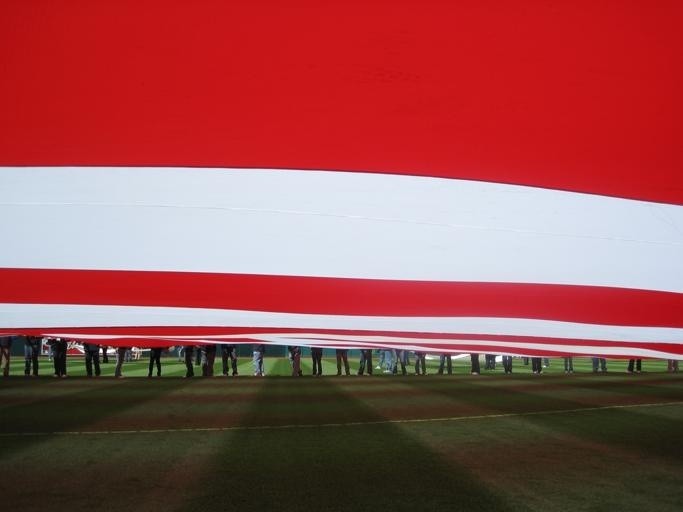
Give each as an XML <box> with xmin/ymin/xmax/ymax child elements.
<box><xmin>3</xmin><ymin>370</ymin><xmax>681</xmax><ymax>379</ymax></box>
